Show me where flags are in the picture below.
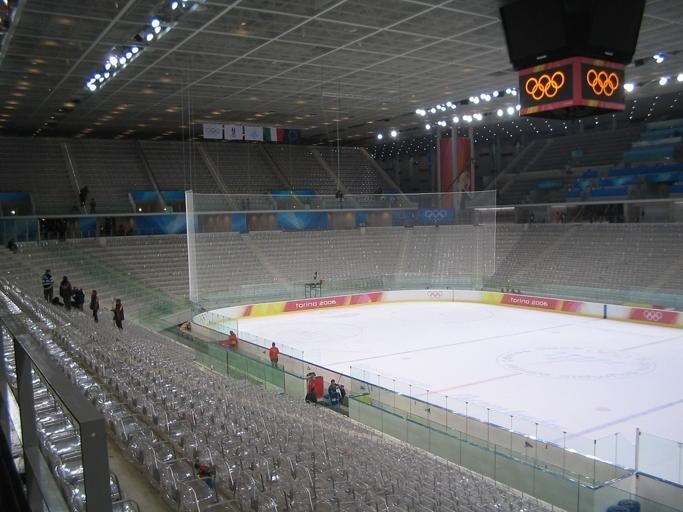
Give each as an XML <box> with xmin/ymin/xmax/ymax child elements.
<box><xmin>244</xmin><ymin>126</ymin><xmax>262</xmax><ymax>141</ymax></box>
<box><xmin>223</xmin><ymin>125</ymin><xmax>243</xmax><ymax>140</ymax></box>
<box><xmin>285</xmin><ymin>129</ymin><xmax>307</xmax><ymax>144</ymax></box>
<box><xmin>203</xmin><ymin>123</ymin><xmax>222</xmax><ymax>139</ymax></box>
<box><xmin>263</xmin><ymin>127</ymin><xmax>283</xmax><ymax>141</ymax></box>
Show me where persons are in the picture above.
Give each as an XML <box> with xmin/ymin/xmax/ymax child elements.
<box><xmin>329</xmin><ymin>385</ymin><xmax>340</xmax><ymax>408</ymax></box>
<box><xmin>528</xmin><ymin>210</ymin><xmax>535</xmax><ymax>223</ymax></box>
<box><xmin>554</xmin><ymin>211</ymin><xmax>560</xmax><ymax>223</ymax></box>
<box><xmin>339</xmin><ymin>384</ymin><xmax>345</xmax><ymax>400</ymax></box>
<box><xmin>227</xmin><ymin>330</ymin><xmax>239</xmax><ymax>353</ymax></box>
<box><xmin>5</xmin><ymin>183</ymin><xmax>134</xmax><ymax>254</ymax></box>
<box><xmin>560</xmin><ymin>211</ymin><xmax>567</xmax><ymax>223</ymax></box>
<box><xmin>268</xmin><ymin>342</ymin><xmax>281</xmax><ymax>368</ymax></box>
<box><xmin>327</xmin><ymin>379</ymin><xmax>337</xmax><ymax>402</ymax></box>
<box><xmin>304</xmin><ymin>385</ymin><xmax>317</xmax><ymax>403</ymax></box>
<box><xmin>335</xmin><ymin>384</ymin><xmax>343</xmax><ymax>405</ymax></box>
<box><xmin>39</xmin><ymin>268</ymin><xmax>126</xmax><ymax>331</ymax></box>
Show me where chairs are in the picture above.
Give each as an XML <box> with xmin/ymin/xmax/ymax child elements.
<box><xmin>0</xmin><ymin>135</ymin><xmax>683</xmax><ymax>512</ymax></box>
